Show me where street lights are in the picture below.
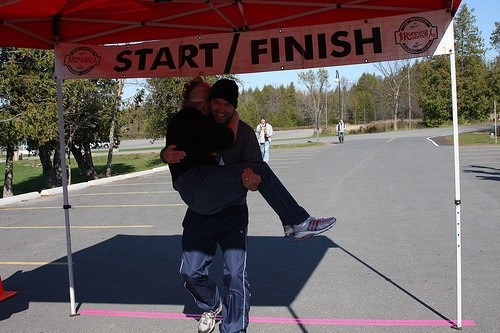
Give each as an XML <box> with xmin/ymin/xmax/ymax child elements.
<box><xmin>335</xmin><ymin>70</ymin><xmax>341</xmax><ymax>120</ymax></box>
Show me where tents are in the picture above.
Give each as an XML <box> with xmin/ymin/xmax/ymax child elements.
<box><xmin>0</xmin><ymin>0</ymin><xmax>463</xmax><ymax>330</ymax></box>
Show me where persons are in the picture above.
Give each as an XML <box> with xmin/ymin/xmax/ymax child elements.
<box><xmin>489</xmin><ymin>112</ymin><xmax>493</xmax><ymax>124</ymax></box>
<box><xmin>166</xmin><ymin>78</ymin><xmax>336</xmax><ymax>241</ymax></box>
<box><xmin>336</xmin><ymin>118</ymin><xmax>346</xmax><ymax>144</ymax></box>
<box><xmin>160</xmin><ymin>80</ymin><xmax>264</xmax><ymax>333</ymax></box>
<box><xmin>256</xmin><ymin>117</ymin><xmax>273</xmax><ymax>164</ymax></box>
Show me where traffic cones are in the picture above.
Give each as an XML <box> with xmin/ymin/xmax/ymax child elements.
<box><xmin>0</xmin><ymin>275</ymin><xmax>17</xmax><ymax>302</ymax></box>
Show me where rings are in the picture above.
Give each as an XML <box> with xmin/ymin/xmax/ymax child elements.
<box><xmin>245</xmin><ymin>177</ymin><xmax>249</xmax><ymax>181</ymax></box>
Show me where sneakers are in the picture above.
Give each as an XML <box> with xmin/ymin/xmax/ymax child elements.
<box><xmin>198</xmin><ymin>296</ymin><xmax>222</xmax><ymax>333</ymax></box>
<box><xmin>283</xmin><ymin>225</ymin><xmax>295</xmax><ymax>238</ymax></box>
<box><xmin>294</xmin><ymin>217</ymin><xmax>336</xmax><ymax>241</ymax></box>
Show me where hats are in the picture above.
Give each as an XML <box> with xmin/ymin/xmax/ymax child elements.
<box><xmin>208</xmin><ymin>79</ymin><xmax>239</xmax><ymax>108</ymax></box>
